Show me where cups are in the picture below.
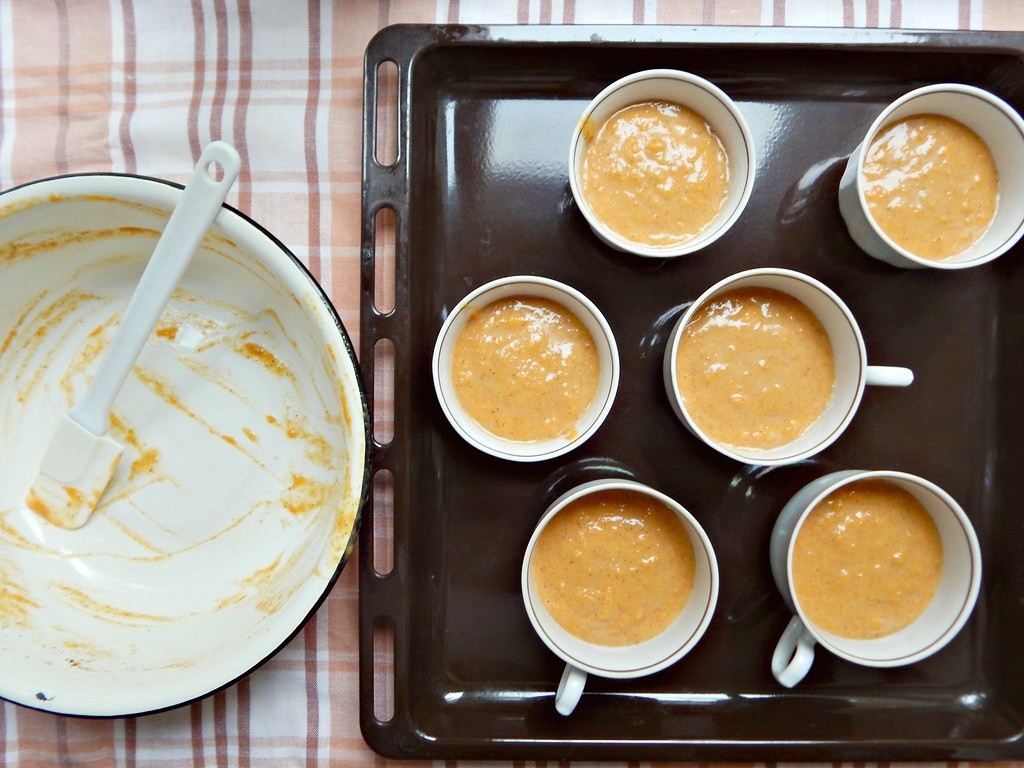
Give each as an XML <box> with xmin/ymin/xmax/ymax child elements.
<box><xmin>515</xmin><ymin>475</ymin><xmax>722</xmax><ymax>718</ymax></box>
<box><xmin>834</xmin><ymin>77</ymin><xmax>1024</xmax><ymax>273</ymax></box>
<box><xmin>764</xmin><ymin>470</ymin><xmax>982</xmax><ymax>690</ymax></box>
<box><xmin>564</xmin><ymin>66</ymin><xmax>756</xmax><ymax>260</ymax></box>
<box><xmin>428</xmin><ymin>274</ymin><xmax>623</xmax><ymax>463</ymax></box>
<box><xmin>661</xmin><ymin>265</ymin><xmax>915</xmax><ymax>466</ymax></box>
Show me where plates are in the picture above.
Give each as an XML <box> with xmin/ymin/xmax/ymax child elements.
<box><xmin>358</xmin><ymin>18</ymin><xmax>1023</xmax><ymax>761</ymax></box>
<box><xmin>1</xmin><ymin>171</ymin><xmax>373</xmax><ymax>719</ymax></box>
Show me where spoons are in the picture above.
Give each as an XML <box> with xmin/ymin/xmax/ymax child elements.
<box><xmin>24</xmin><ymin>137</ymin><xmax>245</xmax><ymax>532</ymax></box>
